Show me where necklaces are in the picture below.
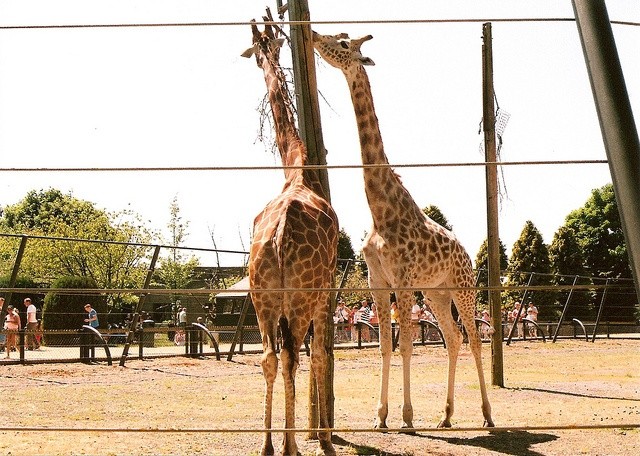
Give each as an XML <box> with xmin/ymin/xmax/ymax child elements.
<box><xmin>175</xmin><ymin>329</ymin><xmax>186</xmax><ymax>346</ymax></box>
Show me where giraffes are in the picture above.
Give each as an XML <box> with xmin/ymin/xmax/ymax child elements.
<box><xmin>310</xmin><ymin>28</ymin><xmax>495</xmax><ymax>435</ymax></box>
<box><xmin>241</xmin><ymin>15</ymin><xmax>340</xmax><ymax>455</ymax></box>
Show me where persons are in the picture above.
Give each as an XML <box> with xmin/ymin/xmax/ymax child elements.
<box><xmin>349</xmin><ymin>306</ymin><xmax>358</xmax><ymax>341</ymax></box>
<box><xmin>513</xmin><ymin>302</ymin><xmax>524</xmax><ymax>338</ymax></box>
<box><xmin>482</xmin><ymin>311</ymin><xmax>490</xmax><ymax>341</ymax></box>
<box><xmin>424</xmin><ymin>300</ymin><xmax>439</xmax><ymax>340</ymax></box>
<box><xmin>3</xmin><ymin>308</ymin><xmax>19</xmax><ymax>329</ymax></box>
<box><xmin>411</xmin><ymin>299</ymin><xmax>421</xmax><ymax>319</ymax></box>
<box><xmin>24</xmin><ymin>298</ymin><xmax>40</xmax><ymax>350</ymax></box>
<box><xmin>84</xmin><ymin>304</ymin><xmax>99</xmax><ymax>328</ymax></box>
<box><xmin>359</xmin><ymin>300</ymin><xmax>370</xmax><ymax>343</ymax></box>
<box><xmin>3</xmin><ymin>305</ymin><xmax>19</xmax><ymax>359</ymax></box>
<box><xmin>526</xmin><ymin>308</ymin><xmax>537</xmax><ymax>338</ymax></box>
<box><xmin>337</xmin><ymin>302</ymin><xmax>351</xmax><ymax>342</ymax></box>
<box><xmin>526</xmin><ymin>302</ymin><xmax>540</xmax><ymax>320</ymax></box>
<box><xmin>507</xmin><ymin>310</ymin><xmax>513</xmax><ymax>338</ymax></box>
<box><xmin>36</xmin><ymin>318</ymin><xmax>41</xmax><ymax>349</ymax></box>
<box><xmin>370</xmin><ymin>304</ymin><xmax>379</xmax><ymax>324</ymax></box>
<box><xmin>177</xmin><ymin>307</ymin><xmax>181</xmax><ymax>325</ymax></box>
<box><xmin>197</xmin><ymin>317</ymin><xmax>205</xmax><ymax>325</ymax></box>
<box><xmin>0</xmin><ymin>297</ymin><xmax>5</xmax><ymax>331</ymax></box>
<box><xmin>180</xmin><ymin>307</ymin><xmax>187</xmax><ymax>327</ymax></box>
<box><xmin>391</xmin><ymin>302</ymin><xmax>400</xmax><ymax>323</ymax></box>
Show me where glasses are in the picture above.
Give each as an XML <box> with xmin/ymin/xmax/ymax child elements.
<box><xmin>529</xmin><ymin>311</ymin><xmax>532</xmax><ymax>313</ymax></box>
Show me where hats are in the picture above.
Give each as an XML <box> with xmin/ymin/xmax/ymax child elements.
<box><xmin>528</xmin><ymin>307</ymin><xmax>533</xmax><ymax>313</ymax></box>
